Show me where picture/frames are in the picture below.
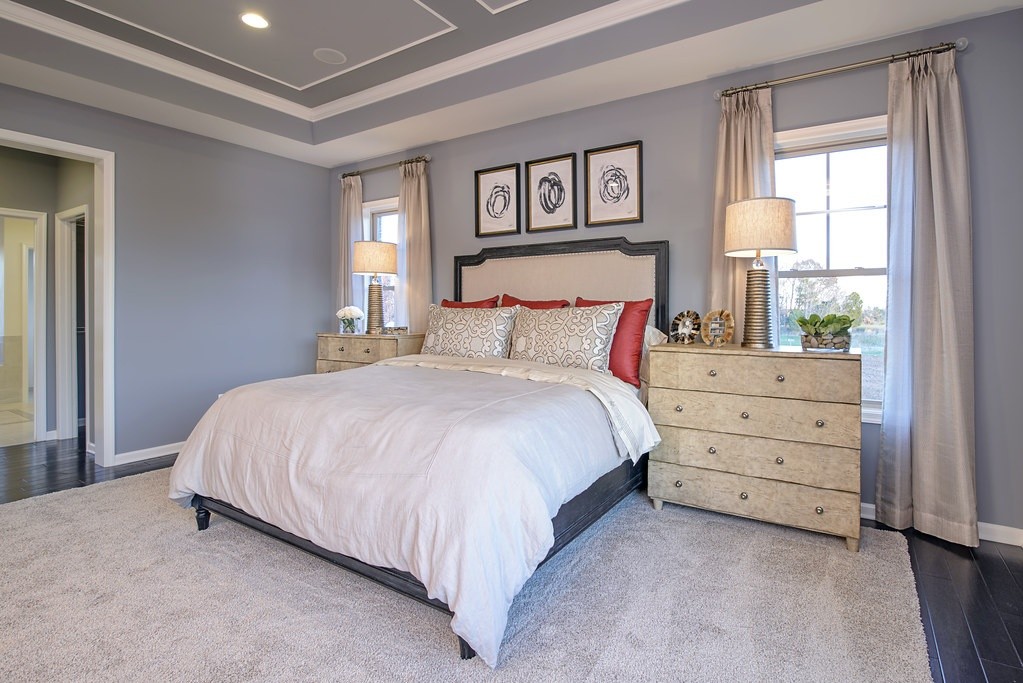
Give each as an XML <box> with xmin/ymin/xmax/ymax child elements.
<box><xmin>525</xmin><ymin>152</ymin><xmax>577</xmax><ymax>234</ymax></box>
<box><xmin>584</xmin><ymin>140</ymin><xmax>644</xmax><ymax>229</ymax></box>
<box><xmin>474</xmin><ymin>163</ymin><xmax>521</xmax><ymax>238</ymax></box>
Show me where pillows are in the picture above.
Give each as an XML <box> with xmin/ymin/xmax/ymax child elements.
<box><xmin>575</xmin><ymin>296</ymin><xmax>654</xmax><ymax>389</ymax></box>
<box><xmin>501</xmin><ymin>294</ymin><xmax>570</xmax><ymax>310</ymax></box>
<box><xmin>441</xmin><ymin>295</ymin><xmax>500</xmax><ymax>309</ymax></box>
<box><xmin>509</xmin><ymin>302</ymin><xmax>625</xmax><ymax>375</ymax></box>
<box><xmin>420</xmin><ymin>304</ymin><xmax>520</xmax><ymax>359</ymax></box>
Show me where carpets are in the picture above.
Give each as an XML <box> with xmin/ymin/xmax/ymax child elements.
<box><xmin>0</xmin><ymin>466</ymin><xmax>934</xmax><ymax>683</ymax></box>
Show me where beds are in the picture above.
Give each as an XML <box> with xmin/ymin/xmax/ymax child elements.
<box><xmin>167</xmin><ymin>237</ymin><xmax>669</xmax><ymax>669</ymax></box>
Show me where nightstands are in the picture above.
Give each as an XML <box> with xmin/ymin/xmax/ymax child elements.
<box><xmin>315</xmin><ymin>332</ymin><xmax>425</xmax><ymax>374</ymax></box>
<box><xmin>649</xmin><ymin>342</ymin><xmax>863</xmax><ymax>553</ymax></box>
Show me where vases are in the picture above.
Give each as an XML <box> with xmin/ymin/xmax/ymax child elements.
<box><xmin>343</xmin><ymin>319</ymin><xmax>355</xmax><ymax>333</ymax></box>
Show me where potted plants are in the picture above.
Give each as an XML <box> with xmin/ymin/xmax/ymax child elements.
<box><xmin>797</xmin><ymin>314</ymin><xmax>856</xmax><ymax>352</ymax></box>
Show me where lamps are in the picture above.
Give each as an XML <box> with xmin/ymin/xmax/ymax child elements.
<box><xmin>352</xmin><ymin>240</ymin><xmax>399</xmax><ymax>334</ymax></box>
<box><xmin>724</xmin><ymin>197</ymin><xmax>798</xmax><ymax>348</ymax></box>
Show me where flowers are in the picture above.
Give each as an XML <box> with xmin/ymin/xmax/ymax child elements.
<box><xmin>336</xmin><ymin>306</ymin><xmax>364</xmax><ymax>321</ymax></box>
